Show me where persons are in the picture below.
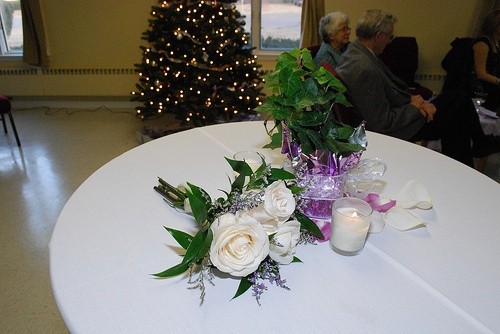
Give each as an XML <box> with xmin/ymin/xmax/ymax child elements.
<box><xmin>334</xmin><ymin>9</ymin><xmax>475</xmax><ymax>172</ymax></box>
<box><xmin>308</xmin><ymin>11</ymin><xmax>352</xmax><ymax>79</ymax></box>
<box><xmin>473</xmin><ymin>9</ymin><xmax>500</xmax><ymax>109</ymax></box>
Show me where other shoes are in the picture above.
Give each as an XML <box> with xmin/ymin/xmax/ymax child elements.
<box><xmin>473</xmin><ymin>136</ymin><xmax>499</xmax><ymax>157</ymax></box>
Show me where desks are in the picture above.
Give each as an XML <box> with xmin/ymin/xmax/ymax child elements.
<box><xmin>45</xmin><ymin>120</ymin><xmax>500</xmax><ymax>334</ymax></box>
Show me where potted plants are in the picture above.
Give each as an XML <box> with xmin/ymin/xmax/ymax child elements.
<box><xmin>254</xmin><ymin>47</ymin><xmax>387</xmax><ymax>220</ymax></box>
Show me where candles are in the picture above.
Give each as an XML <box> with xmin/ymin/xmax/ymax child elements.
<box><xmin>332</xmin><ymin>196</ymin><xmax>373</xmax><ymax>256</ymax></box>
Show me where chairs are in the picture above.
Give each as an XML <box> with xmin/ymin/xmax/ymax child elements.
<box><xmin>441</xmin><ymin>36</ymin><xmax>477</xmax><ymax>91</ymax></box>
<box><xmin>298</xmin><ymin>44</ymin><xmax>321</xmax><ymax>79</ymax></box>
<box><xmin>332</xmin><ymin>101</ymin><xmax>362</xmax><ymax>131</ymax></box>
<box><xmin>383</xmin><ymin>36</ymin><xmax>433</xmax><ymax>101</ymax></box>
<box><xmin>0</xmin><ymin>95</ymin><xmax>22</xmax><ymax>148</ymax></box>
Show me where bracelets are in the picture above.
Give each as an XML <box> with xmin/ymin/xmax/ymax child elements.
<box><xmin>496</xmin><ymin>78</ymin><xmax>500</xmax><ymax>85</ymax></box>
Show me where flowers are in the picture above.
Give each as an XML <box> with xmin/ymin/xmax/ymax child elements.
<box><xmin>148</xmin><ymin>152</ymin><xmax>325</xmax><ymax>307</ymax></box>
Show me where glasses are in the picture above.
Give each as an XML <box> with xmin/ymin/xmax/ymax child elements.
<box><xmin>336</xmin><ymin>26</ymin><xmax>351</xmax><ymax>33</ymax></box>
<box><xmin>382</xmin><ymin>32</ymin><xmax>395</xmax><ymax>41</ymax></box>
